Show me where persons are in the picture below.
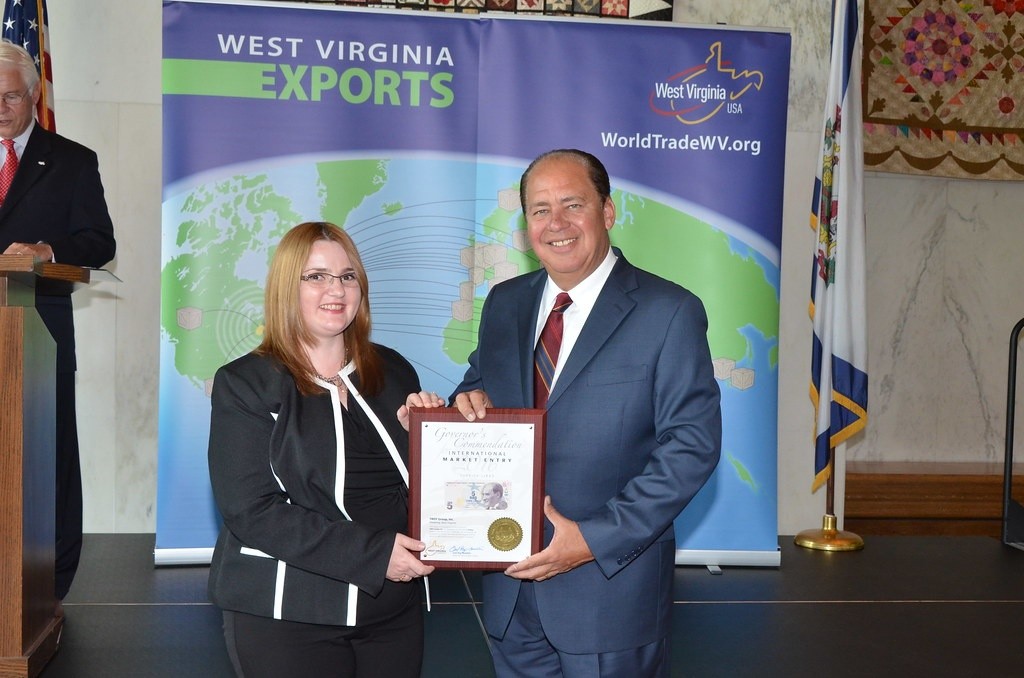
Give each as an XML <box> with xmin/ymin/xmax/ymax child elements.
<box><xmin>0</xmin><ymin>37</ymin><xmax>117</xmax><ymax>605</ymax></box>
<box><xmin>207</xmin><ymin>221</ymin><xmax>446</xmax><ymax>678</ymax></box>
<box><xmin>447</xmin><ymin>146</ymin><xmax>724</xmax><ymax>678</ymax></box>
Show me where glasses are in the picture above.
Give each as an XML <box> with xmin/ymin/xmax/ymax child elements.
<box><xmin>300</xmin><ymin>272</ymin><xmax>360</xmax><ymax>289</ymax></box>
<box><xmin>0</xmin><ymin>87</ymin><xmax>28</xmax><ymax>105</ymax></box>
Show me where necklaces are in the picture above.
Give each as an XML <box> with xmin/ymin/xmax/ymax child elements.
<box><xmin>301</xmin><ymin>346</ymin><xmax>351</xmax><ymax>394</ymax></box>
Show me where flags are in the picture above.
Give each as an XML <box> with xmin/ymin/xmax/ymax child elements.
<box><xmin>808</xmin><ymin>0</ymin><xmax>869</xmax><ymax>496</ymax></box>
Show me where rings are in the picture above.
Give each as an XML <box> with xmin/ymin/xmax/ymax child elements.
<box><xmin>401</xmin><ymin>573</ymin><xmax>407</xmax><ymax>583</ymax></box>
<box><xmin>16</xmin><ymin>251</ymin><xmax>24</xmax><ymax>256</ymax></box>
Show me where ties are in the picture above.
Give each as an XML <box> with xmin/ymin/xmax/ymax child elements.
<box><xmin>532</xmin><ymin>293</ymin><xmax>573</xmax><ymax>409</ymax></box>
<box><xmin>0</xmin><ymin>139</ymin><xmax>20</xmax><ymax>207</ymax></box>
